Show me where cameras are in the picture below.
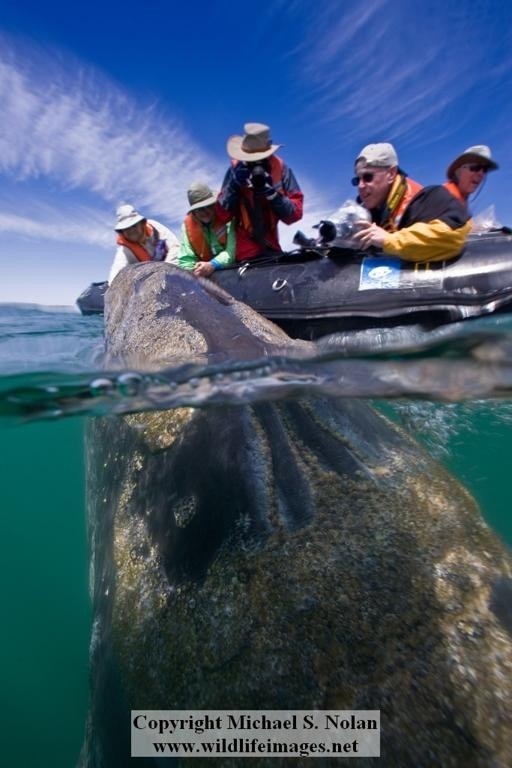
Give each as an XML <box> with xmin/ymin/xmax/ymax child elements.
<box><xmin>292</xmin><ymin>231</ymin><xmax>315</xmax><ymax>249</ymax></box>
<box><xmin>245</xmin><ymin>160</ymin><xmax>270</xmax><ymax>189</ymax></box>
<box><xmin>314</xmin><ymin>218</ymin><xmax>356</xmax><ymax>241</ymax></box>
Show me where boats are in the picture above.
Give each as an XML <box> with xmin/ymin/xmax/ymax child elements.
<box><xmin>76</xmin><ymin>230</ymin><xmax>512</xmax><ymax>340</ymax></box>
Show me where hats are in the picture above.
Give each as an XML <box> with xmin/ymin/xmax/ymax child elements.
<box><xmin>186</xmin><ymin>182</ymin><xmax>219</xmax><ymax>215</ymax></box>
<box><xmin>226</xmin><ymin>123</ymin><xmax>284</xmax><ymax>162</ymax></box>
<box><xmin>447</xmin><ymin>145</ymin><xmax>499</xmax><ymax>179</ymax></box>
<box><xmin>114</xmin><ymin>205</ymin><xmax>146</xmax><ymax>231</ymax></box>
<box><xmin>354</xmin><ymin>143</ymin><xmax>408</xmax><ymax>177</ymax></box>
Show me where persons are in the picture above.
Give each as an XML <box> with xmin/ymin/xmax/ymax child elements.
<box><xmin>219</xmin><ymin>121</ymin><xmax>303</xmax><ymax>262</ymax></box>
<box><xmin>108</xmin><ymin>205</ymin><xmax>180</xmax><ymax>288</ymax></box>
<box><xmin>301</xmin><ymin>141</ymin><xmax>473</xmax><ymax>263</ymax></box>
<box><xmin>440</xmin><ymin>144</ymin><xmax>498</xmax><ymax>233</ymax></box>
<box><xmin>177</xmin><ymin>183</ymin><xmax>237</xmax><ymax>278</ymax></box>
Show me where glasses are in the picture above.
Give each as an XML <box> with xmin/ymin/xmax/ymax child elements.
<box><xmin>351</xmin><ymin>173</ymin><xmax>373</xmax><ymax>186</ymax></box>
<box><xmin>470</xmin><ymin>165</ymin><xmax>488</xmax><ymax>173</ymax></box>
